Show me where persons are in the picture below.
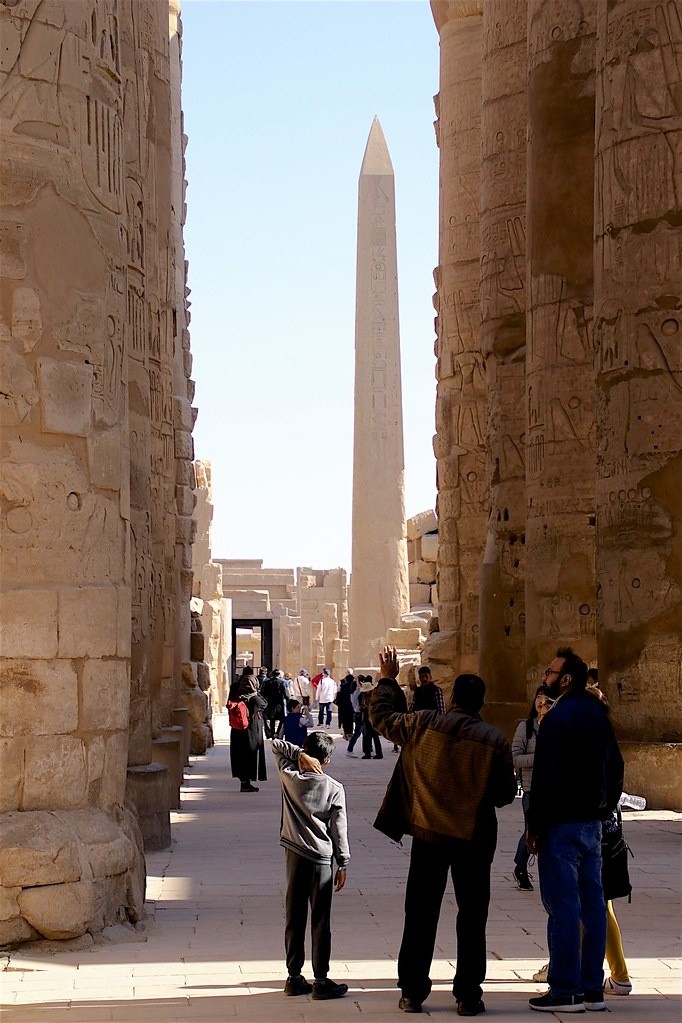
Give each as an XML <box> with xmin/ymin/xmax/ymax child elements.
<box><xmin>512</xmin><ymin>646</ymin><xmax>632</xmax><ymax>1014</ymax></box>
<box><xmin>367</xmin><ymin>647</ymin><xmax>517</xmax><ymax>1016</ymax></box>
<box><xmin>228</xmin><ymin>665</ymin><xmax>337</xmax><ymax>793</ymax></box>
<box><xmin>333</xmin><ymin>668</ymin><xmax>383</xmax><ymax>760</ymax></box>
<box><xmin>408</xmin><ymin>667</ymin><xmax>446</xmax><ymax>715</ymax></box>
<box><xmin>271</xmin><ymin>731</ymin><xmax>351</xmax><ymax>1000</ymax></box>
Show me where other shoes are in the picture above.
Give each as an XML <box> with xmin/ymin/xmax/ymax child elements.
<box><xmin>398</xmin><ymin>996</ymin><xmax>422</xmax><ymax>1013</ymax></box>
<box><xmin>241</xmin><ymin>782</ymin><xmax>259</xmax><ymax>792</ymax></box>
<box><xmin>318</xmin><ymin>723</ymin><xmax>324</xmax><ymax>726</ymax></box>
<box><xmin>340</xmin><ymin>730</ymin><xmax>345</xmax><ymax>735</ymax></box>
<box><xmin>373</xmin><ymin>755</ymin><xmax>383</xmax><ymax>759</ymax></box>
<box><xmin>362</xmin><ymin>754</ymin><xmax>371</xmax><ymax>759</ymax></box>
<box><xmin>370</xmin><ymin>751</ymin><xmax>376</xmax><ymax>756</ymax></box>
<box><xmin>347</xmin><ymin>734</ymin><xmax>353</xmax><ymax>737</ymax></box>
<box><xmin>343</xmin><ymin>736</ymin><xmax>349</xmax><ymax>741</ymax></box>
<box><xmin>325</xmin><ymin>724</ymin><xmax>329</xmax><ymax>728</ymax></box>
<box><xmin>456</xmin><ymin>998</ymin><xmax>485</xmax><ymax>1015</ymax></box>
<box><xmin>346</xmin><ymin>749</ymin><xmax>358</xmax><ymax>757</ymax></box>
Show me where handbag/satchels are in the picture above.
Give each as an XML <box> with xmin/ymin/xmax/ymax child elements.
<box><xmin>303</xmin><ymin>697</ymin><xmax>310</xmax><ymax>705</ymax></box>
<box><xmin>601</xmin><ymin>829</ymin><xmax>633</xmax><ymax>900</ymax></box>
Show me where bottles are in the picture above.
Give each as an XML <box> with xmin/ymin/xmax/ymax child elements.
<box><xmin>619</xmin><ymin>792</ymin><xmax>646</xmax><ymax>810</ymax></box>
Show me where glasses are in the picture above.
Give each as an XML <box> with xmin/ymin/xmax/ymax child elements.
<box><xmin>545</xmin><ymin>668</ymin><xmax>565</xmax><ymax>677</ymax></box>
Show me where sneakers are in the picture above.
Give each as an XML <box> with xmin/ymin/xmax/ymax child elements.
<box><xmin>312</xmin><ymin>980</ymin><xmax>348</xmax><ymax>999</ymax></box>
<box><xmin>533</xmin><ymin>963</ymin><xmax>549</xmax><ymax>982</ymax></box>
<box><xmin>582</xmin><ymin>987</ymin><xmax>605</xmax><ymax>1009</ymax></box>
<box><xmin>284</xmin><ymin>976</ymin><xmax>314</xmax><ymax>995</ymax></box>
<box><xmin>601</xmin><ymin>977</ymin><xmax>632</xmax><ymax>995</ymax></box>
<box><xmin>513</xmin><ymin>867</ymin><xmax>534</xmax><ymax>891</ymax></box>
<box><xmin>528</xmin><ymin>989</ymin><xmax>585</xmax><ymax>1013</ymax></box>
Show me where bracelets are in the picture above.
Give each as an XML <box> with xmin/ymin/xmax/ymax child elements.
<box><xmin>339</xmin><ymin>867</ymin><xmax>346</xmax><ymax>872</ymax></box>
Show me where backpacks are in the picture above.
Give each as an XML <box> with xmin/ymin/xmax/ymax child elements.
<box><xmin>226</xmin><ymin>695</ymin><xmax>257</xmax><ymax>731</ymax></box>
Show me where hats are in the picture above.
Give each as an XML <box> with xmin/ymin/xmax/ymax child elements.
<box><xmin>300</xmin><ymin>669</ymin><xmax>307</xmax><ymax>674</ymax></box>
<box><xmin>323</xmin><ymin>670</ymin><xmax>330</xmax><ymax>675</ymax></box>
<box><xmin>359</xmin><ymin>682</ymin><xmax>375</xmax><ymax>692</ymax></box>
